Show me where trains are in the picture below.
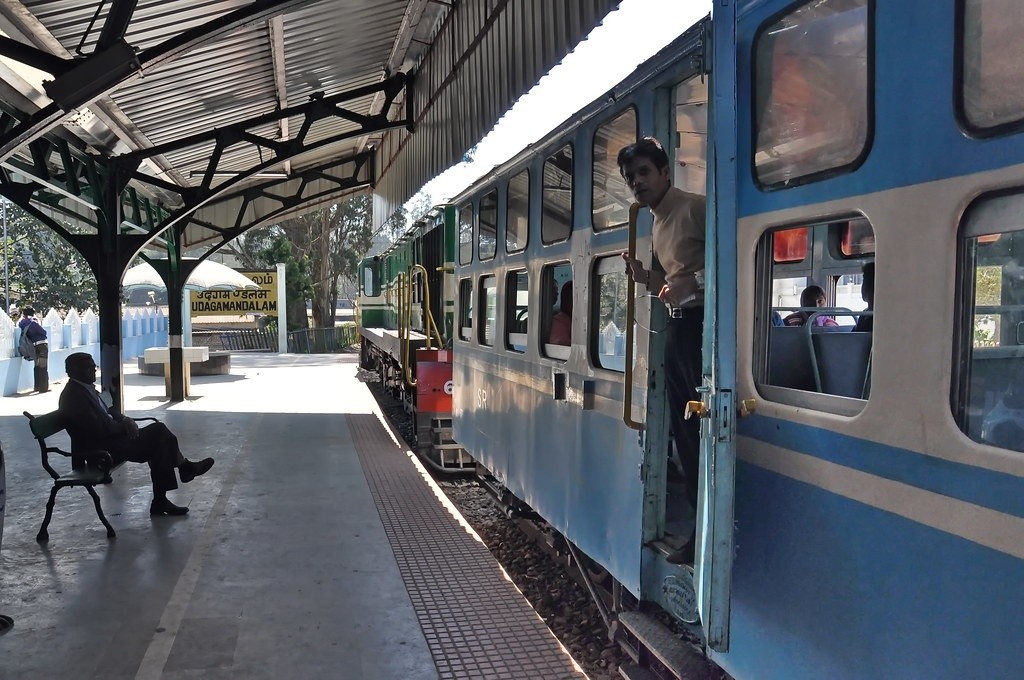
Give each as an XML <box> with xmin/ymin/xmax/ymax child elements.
<box><xmin>359</xmin><ymin>0</ymin><xmax>1024</xmax><ymax>680</ymax></box>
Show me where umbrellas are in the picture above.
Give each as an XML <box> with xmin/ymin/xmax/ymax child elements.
<box><xmin>121</xmin><ymin>257</ymin><xmax>263</xmax><ymax>348</ymax></box>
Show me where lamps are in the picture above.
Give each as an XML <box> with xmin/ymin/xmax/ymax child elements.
<box><xmin>190</xmin><ymin>170</ymin><xmax>289</xmax><ymax>180</ymax></box>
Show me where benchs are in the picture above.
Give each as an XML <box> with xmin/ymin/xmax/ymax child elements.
<box><xmin>23</xmin><ymin>389</ymin><xmax>159</xmax><ymax>541</ymax></box>
<box><xmin>769</xmin><ymin>307</ymin><xmax>1024</xmax><ymax>451</ymax></box>
<box><xmin>516</xmin><ymin>309</ymin><xmax>528</xmax><ymax>333</ymax></box>
<box><xmin>137</xmin><ymin>352</ymin><xmax>231</xmax><ymax>376</ymax></box>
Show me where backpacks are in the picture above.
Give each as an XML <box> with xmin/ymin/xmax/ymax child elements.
<box><xmin>17</xmin><ymin>323</ymin><xmax>36</xmax><ymax>361</ymax></box>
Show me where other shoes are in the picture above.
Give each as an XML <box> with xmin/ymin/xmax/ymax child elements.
<box><xmin>179</xmin><ymin>457</ymin><xmax>214</xmax><ymax>483</ymax></box>
<box><xmin>667</xmin><ymin>540</ymin><xmax>695</xmax><ymax>564</ymax></box>
<box><xmin>149</xmin><ymin>497</ymin><xmax>189</xmax><ymax>516</ymax></box>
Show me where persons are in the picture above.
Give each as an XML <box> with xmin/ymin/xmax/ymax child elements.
<box><xmin>616</xmin><ymin>136</ymin><xmax>706</xmax><ymax>567</ymax></box>
<box><xmin>549</xmin><ymin>279</ymin><xmax>573</xmax><ymax>348</ymax></box>
<box><xmin>58</xmin><ymin>352</ymin><xmax>215</xmax><ymax>515</ymax></box>
<box><xmin>0</xmin><ymin>440</ymin><xmax>14</xmax><ymax>631</ymax></box>
<box><xmin>19</xmin><ymin>305</ymin><xmax>52</xmax><ymax>393</ymax></box>
<box><xmin>599</xmin><ymin>308</ymin><xmax>621</xmax><ymax>356</ymax></box>
<box><xmin>771</xmin><ymin>261</ymin><xmax>875</xmax><ymax>332</ymax></box>
